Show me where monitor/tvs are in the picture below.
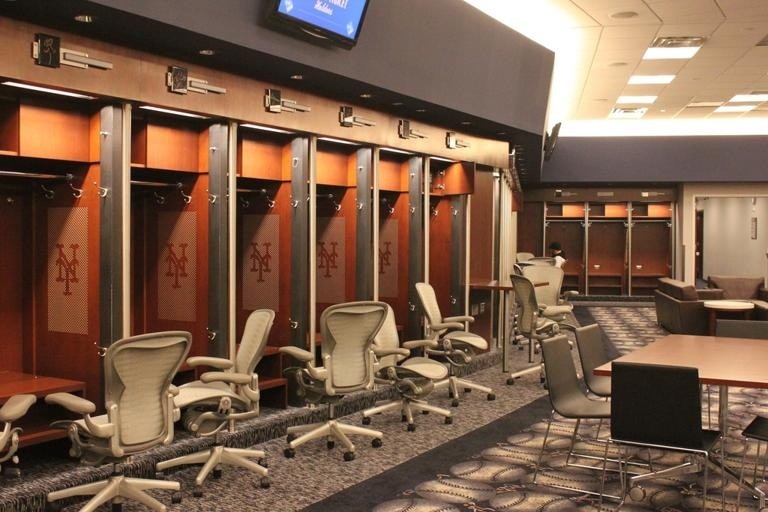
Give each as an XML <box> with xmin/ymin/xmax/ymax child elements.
<box><xmin>260</xmin><ymin>0</ymin><xmax>370</xmax><ymax>52</ymax></box>
<box><xmin>544</xmin><ymin>122</ymin><xmax>561</xmax><ymax>161</ymax></box>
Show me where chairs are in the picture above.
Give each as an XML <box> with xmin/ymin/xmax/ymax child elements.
<box><xmin>513</xmin><ymin>265</ymin><xmax>574</xmax><ymax>367</ymax></box>
<box><xmin>280</xmin><ymin>302</ymin><xmax>389</xmax><ymax>461</ymax></box>
<box><xmin>528</xmin><ymin>257</ymin><xmax>555</xmax><ymax>267</ymax></box>
<box><xmin>414</xmin><ymin>283</ymin><xmax>495</xmax><ymax>408</ymax></box>
<box><xmin>533</xmin><ymin>336</ymin><xmax>651</xmax><ymax>498</ymax></box>
<box><xmin>506</xmin><ymin>275</ymin><xmax>577</xmax><ymax>385</ymax></box>
<box><xmin>736</xmin><ymin>415</ymin><xmax>768</xmax><ymax>512</ymax></box>
<box><xmin>362</xmin><ymin>302</ymin><xmax>454</xmax><ymax>430</ymax></box>
<box><xmin>597</xmin><ymin>362</ymin><xmax>749</xmax><ymax>512</ymax></box>
<box><xmin>516</xmin><ymin>252</ymin><xmax>534</xmax><ymax>263</ymax></box>
<box><xmin>0</xmin><ymin>393</ymin><xmax>41</xmax><ymax>476</ymax></box>
<box><xmin>46</xmin><ymin>331</ymin><xmax>181</xmax><ymax>512</ymax></box>
<box><xmin>575</xmin><ymin>323</ymin><xmax>661</xmax><ymax>476</ymax></box>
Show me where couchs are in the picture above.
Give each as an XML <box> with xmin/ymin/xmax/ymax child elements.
<box><xmin>654</xmin><ymin>277</ymin><xmax>724</xmax><ymax>334</ymax></box>
<box><xmin>703</xmin><ymin>276</ymin><xmax>768</xmax><ymax>338</ymax></box>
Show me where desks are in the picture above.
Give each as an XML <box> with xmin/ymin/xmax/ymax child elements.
<box><xmin>471</xmin><ymin>281</ymin><xmax>549</xmax><ymax>361</ymax></box>
<box><xmin>0</xmin><ymin>372</ymin><xmax>86</xmax><ymax>473</ymax></box>
<box><xmin>592</xmin><ymin>334</ymin><xmax>768</xmax><ymax>492</ymax></box>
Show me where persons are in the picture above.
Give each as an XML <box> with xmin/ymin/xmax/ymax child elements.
<box><xmin>549</xmin><ymin>241</ymin><xmax>567</xmax><ymax>269</ymax></box>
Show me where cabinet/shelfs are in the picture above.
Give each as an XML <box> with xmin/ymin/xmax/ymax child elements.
<box><xmin>154</xmin><ymin>309</ymin><xmax>275</xmax><ymax>496</ymax></box>
